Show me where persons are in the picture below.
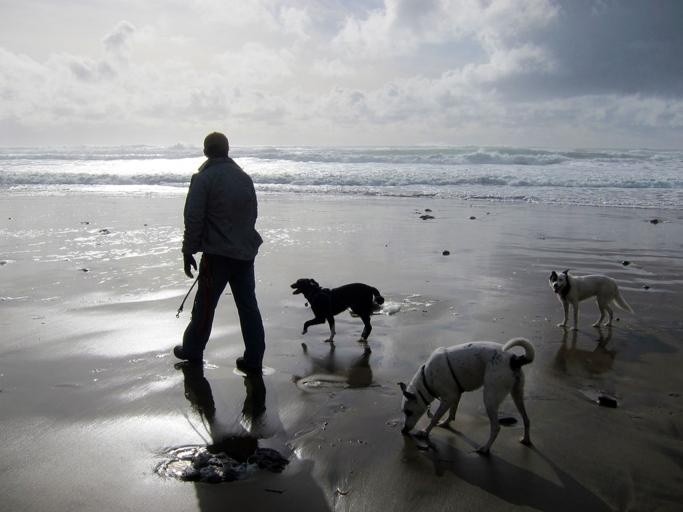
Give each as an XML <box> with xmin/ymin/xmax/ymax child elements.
<box><xmin>172</xmin><ymin>132</ymin><xmax>265</xmax><ymax>374</ymax></box>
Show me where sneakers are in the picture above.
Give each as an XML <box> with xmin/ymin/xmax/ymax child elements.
<box><xmin>174</xmin><ymin>346</ymin><xmax>203</xmax><ymax>361</ymax></box>
<box><xmin>236</xmin><ymin>358</ymin><xmax>263</xmax><ymax>372</ymax></box>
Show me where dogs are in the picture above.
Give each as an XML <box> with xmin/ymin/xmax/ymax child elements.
<box><xmin>291</xmin><ymin>278</ymin><xmax>385</xmax><ymax>344</ymax></box>
<box><xmin>549</xmin><ymin>268</ymin><xmax>635</xmax><ymax>332</ymax></box>
<box><xmin>396</xmin><ymin>336</ymin><xmax>536</xmax><ymax>457</ymax></box>
